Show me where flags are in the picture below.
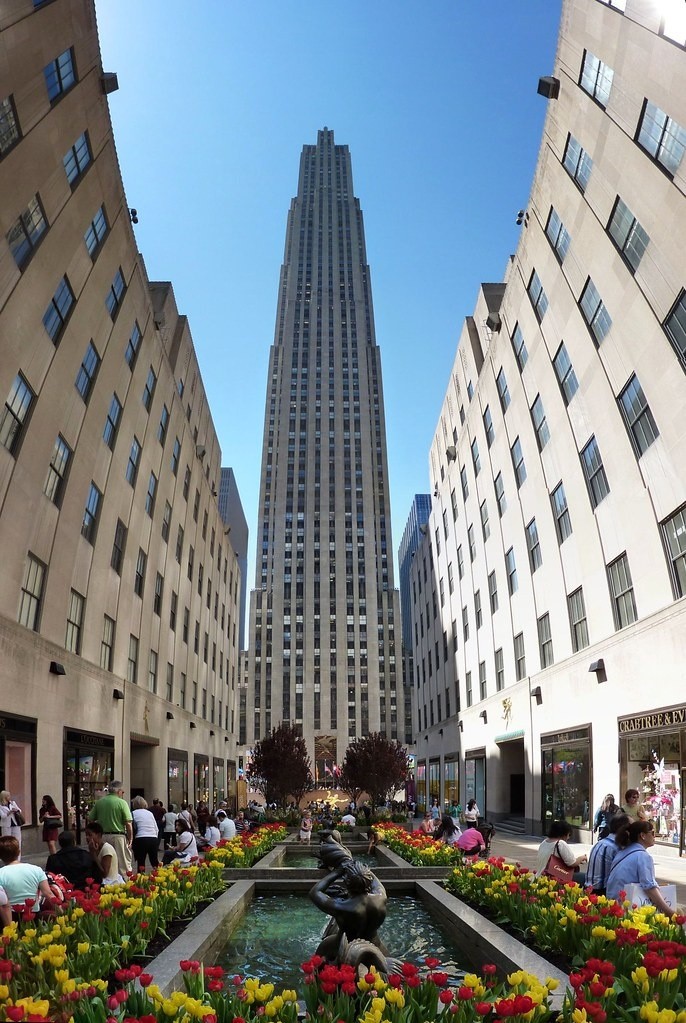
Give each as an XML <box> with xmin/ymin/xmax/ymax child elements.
<box><xmin>326</xmin><ymin>765</ymin><xmax>333</xmax><ymax>777</ymax></box>
<box><xmin>334</xmin><ymin>765</ymin><xmax>341</xmax><ymax>777</ymax></box>
<box><xmin>316</xmin><ymin>767</ymin><xmax>319</xmax><ymax>782</ymax></box>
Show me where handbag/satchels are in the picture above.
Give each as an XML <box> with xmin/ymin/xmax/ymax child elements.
<box><xmin>623</xmin><ymin>883</ymin><xmax>677</xmax><ymax>918</ymax></box>
<box><xmin>131</xmin><ymin>812</ymin><xmax>138</xmax><ymax>838</ymax></box>
<box><xmin>162</xmin><ymin>815</ymin><xmax>166</xmax><ymax>829</ymax></box>
<box><xmin>46</xmin><ymin>818</ymin><xmax>62</xmax><ymax>828</ymax></box>
<box><xmin>15</xmin><ymin>811</ymin><xmax>24</xmax><ymax>826</ymax></box>
<box><xmin>544</xmin><ymin>840</ymin><xmax>575</xmax><ymax>882</ymax></box>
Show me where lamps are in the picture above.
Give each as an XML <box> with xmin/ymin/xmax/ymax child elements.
<box><xmin>128</xmin><ymin>207</ymin><xmax>138</xmax><ymax>225</ymax></box>
<box><xmin>531</xmin><ymin>686</ymin><xmax>543</xmax><ymax>706</ymax></box>
<box><xmin>589</xmin><ymin>659</ymin><xmax>606</xmax><ymax>683</ymax></box>
<box><xmin>480</xmin><ymin>710</ymin><xmax>487</xmax><ymax>724</ymax></box>
<box><xmin>167</xmin><ymin>712</ymin><xmax>174</xmax><ymax>719</ymax></box>
<box><xmin>413</xmin><ymin>740</ymin><xmax>416</xmax><ymax>744</ymax></box>
<box><xmin>210</xmin><ymin>730</ymin><xmax>215</xmax><ymax>736</ymax></box>
<box><xmin>516</xmin><ymin>210</ymin><xmax>527</xmax><ymax>226</ymax></box>
<box><xmin>237</xmin><ymin>742</ymin><xmax>239</xmax><ymax>746</ymax></box>
<box><xmin>49</xmin><ymin>662</ymin><xmax>66</xmax><ymax>675</ymax></box>
<box><xmin>457</xmin><ymin>720</ymin><xmax>463</xmax><ymax>732</ymax></box>
<box><xmin>438</xmin><ymin>729</ymin><xmax>443</xmax><ymax>735</ymax></box>
<box><xmin>225</xmin><ymin>737</ymin><xmax>229</xmax><ymax>741</ymax></box>
<box><xmin>424</xmin><ymin>735</ymin><xmax>428</xmax><ymax>742</ymax></box>
<box><xmin>113</xmin><ymin>689</ymin><xmax>124</xmax><ymax>699</ymax></box>
<box><xmin>190</xmin><ymin>721</ymin><xmax>197</xmax><ymax>728</ymax></box>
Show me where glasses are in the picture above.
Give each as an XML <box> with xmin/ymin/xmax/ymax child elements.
<box><xmin>631</xmin><ymin>796</ymin><xmax>639</xmax><ymax>798</ymax></box>
<box><xmin>645</xmin><ymin>831</ymin><xmax>655</xmax><ymax>836</ymax></box>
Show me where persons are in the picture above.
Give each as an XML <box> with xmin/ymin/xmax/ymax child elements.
<box><xmin>367</xmin><ymin>829</ymin><xmax>388</xmax><ymax>854</ymax></box>
<box><xmin>311</xmin><ymin>860</ymin><xmax>391</xmax><ymax>974</ymax></box>
<box><xmin>196</xmin><ymin>801</ymin><xmax>250</xmax><ymax>852</ymax></box>
<box><xmin>0</xmin><ymin>791</ymin><xmax>62</xmax><ymax>862</ymax></box>
<box><xmin>584</xmin><ymin>815</ymin><xmax>630</xmax><ymax>896</ymax></box>
<box><xmin>248</xmin><ymin>799</ymin><xmax>264</xmax><ymax>814</ymax></box>
<box><xmin>616</xmin><ymin>789</ymin><xmax>645</xmax><ymax>821</ymax></box>
<box><xmin>536</xmin><ymin>820</ymin><xmax>587</xmax><ymax>888</ymax></box>
<box><xmin>0</xmin><ymin>831</ymin><xmax>103</xmax><ymax>933</ymax></box>
<box><xmin>419</xmin><ymin>797</ymin><xmax>492</xmax><ymax>856</ymax></box>
<box><xmin>299</xmin><ymin>811</ymin><xmax>312</xmax><ymax>845</ymax></box>
<box><xmin>606</xmin><ymin>820</ymin><xmax>684</xmax><ymax>918</ymax></box>
<box><xmin>593</xmin><ymin>794</ymin><xmax>618</xmax><ymax>841</ymax></box>
<box><xmin>269</xmin><ymin>801</ymin><xmax>294</xmax><ymax>811</ymax></box>
<box><xmin>301</xmin><ymin>800</ymin><xmax>356</xmax><ymax>830</ymax></box>
<box><xmin>85</xmin><ymin>781</ymin><xmax>198</xmax><ymax>895</ymax></box>
<box><xmin>385</xmin><ymin>794</ymin><xmax>418</xmax><ymax>822</ymax></box>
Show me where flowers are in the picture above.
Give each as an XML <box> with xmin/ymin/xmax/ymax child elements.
<box><xmin>0</xmin><ymin>810</ymin><xmax>686</xmax><ymax>1023</ymax></box>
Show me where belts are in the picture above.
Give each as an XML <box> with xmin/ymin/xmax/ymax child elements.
<box><xmin>102</xmin><ymin>832</ymin><xmax>125</xmax><ymax>835</ymax></box>
